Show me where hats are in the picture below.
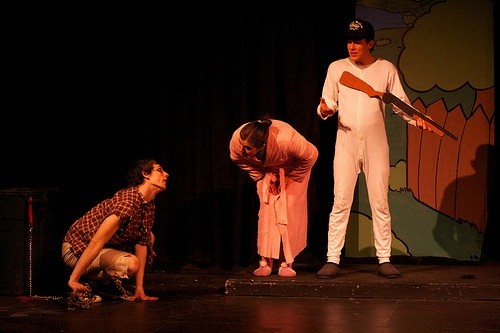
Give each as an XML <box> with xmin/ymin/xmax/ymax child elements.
<box><xmin>339</xmin><ymin>20</ymin><xmax>375</xmax><ymax>42</ymax></box>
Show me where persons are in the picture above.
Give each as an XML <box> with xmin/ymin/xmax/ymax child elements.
<box><xmin>62</xmin><ymin>158</ymin><xmax>171</xmax><ymax>304</ymax></box>
<box><xmin>228</xmin><ymin>115</ymin><xmax>318</xmax><ymax>277</ymax></box>
<box><xmin>316</xmin><ymin>18</ymin><xmax>435</xmax><ymax>278</ymax></box>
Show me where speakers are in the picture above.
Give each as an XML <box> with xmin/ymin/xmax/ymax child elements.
<box><xmin>0</xmin><ymin>194</ymin><xmax>48</xmax><ymax>296</ymax></box>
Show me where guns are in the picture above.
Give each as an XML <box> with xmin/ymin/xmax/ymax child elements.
<box><xmin>339</xmin><ymin>71</ymin><xmax>458</xmax><ymax>141</ymax></box>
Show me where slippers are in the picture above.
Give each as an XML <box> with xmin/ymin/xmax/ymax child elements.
<box><xmin>376</xmin><ymin>261</ymin><xmax>401</xmax><ymax>278</ymax></box>
<box><xmin>252</xmin><ymin>261</ymin><xmax>274</xmax><ymax>277</ymax></box>
<box><xmin>277</xmin><ymin>262</ymin><xmax>297</xmax><ymax>276</ymax></box>
<box><xmin>316</xmin><ymin>262</ymin><xmax>341</xmax><ymax>278</ymax></box>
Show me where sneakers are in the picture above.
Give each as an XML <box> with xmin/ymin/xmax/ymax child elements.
<box><xmin>98</xmin><ymin>276</ymin><xmax>130</xmax><ymax>302</ymax></box>
<box><xmin>77</xmin><ymin>280</ymin><xmax>101</xmax><ymax>303</ymax></box>
